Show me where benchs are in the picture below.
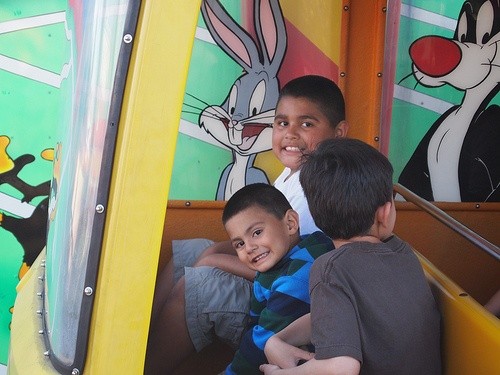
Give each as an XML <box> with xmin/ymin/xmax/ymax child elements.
<box><xmin>164</xmin><ymin>197</ymin><xmax>500</xmax><ymax>375</ymax></box>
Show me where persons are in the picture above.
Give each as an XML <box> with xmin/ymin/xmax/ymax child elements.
<box><xmin>259</xmin><ymin>137</ymin><xmax>442</xmax><ymax>375</ymax></box>
<box><xmin>144</xmin><ymin>75</ymin><xmax>349</xmax><ymax>375</ymax></box>
<box><xmin>218</xmin><ymin>183</ymin><xmax>336</xmax><ymax>375</ymax></box>
